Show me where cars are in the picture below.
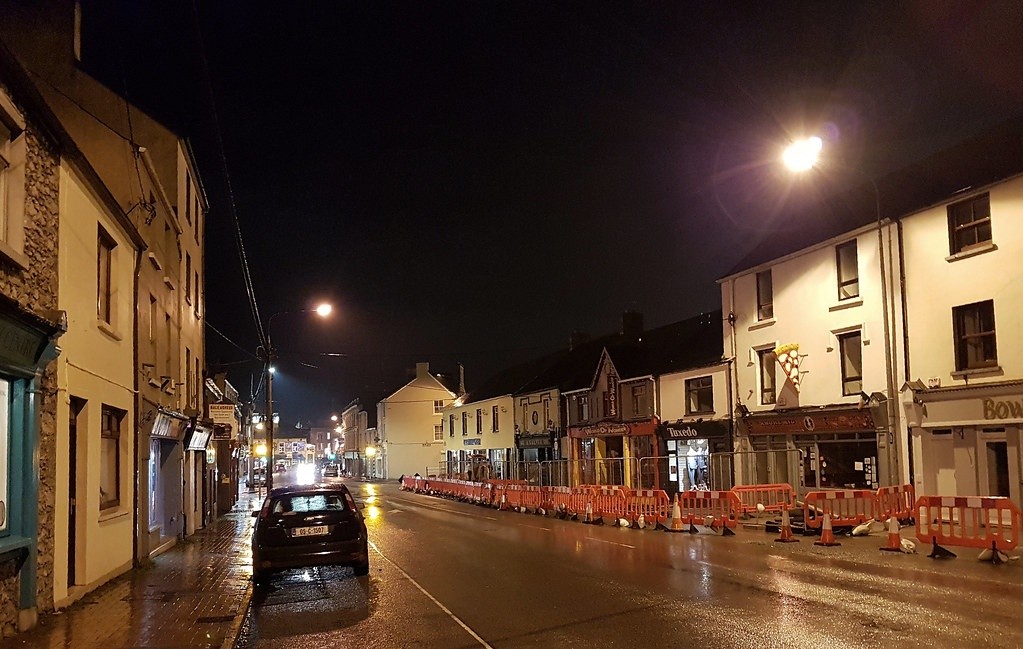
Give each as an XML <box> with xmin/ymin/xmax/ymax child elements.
<box><xmin>274</xmin><ymin>463</ymin><xmax>338</xmax><ymax>476</ymax></box>
<box><xmin>251</xmin><ymin>482</ymin><xmax>370</xmax><ymax>581</ymax></box>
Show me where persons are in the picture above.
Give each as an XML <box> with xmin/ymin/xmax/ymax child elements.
<box><xmin>686</xmin><ymin>448</ymin><xmax>707</xmax><ymax>491</ymax></box>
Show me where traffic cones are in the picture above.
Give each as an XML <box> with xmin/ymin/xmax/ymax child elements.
<box><xmin>879</xmin><ymin>508</ymin><xmax>916</xmax><ymax>553</ymax></box>
<box><xmin>498</xmin><ymin>492</ymin><xmax>509</xmax><ymax>511</ymax></box>
<box><xmin>664</xmin><ymin>492</ymin><xmax>691</xmax><ymax>533</ymax></box>
<box><xmin>813</xmin><ymin>505</ymin><xmax>842</xmax><ymax>547</ymax></box>
<box><xmin>398</xmin><ymin>480</ymin><xmax>421</xmax><ymax>493</ymax></box>
<box><xmin>774</xmin><ymin>501</ymin><xmax>800</xmax><ymax>542</ymax></box>
<box><xmin>582</xmin><ymin>500</ymin><xmax>598</xmax><ymax>523</ymax></box>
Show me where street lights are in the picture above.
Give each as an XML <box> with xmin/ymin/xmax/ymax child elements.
<box><xmin>784</xmin><ymin>138</ymin><xmax>901</xmax><ymax>531</ymax></box>
<box><xmin>264</xmin><ymin>302</ymin><xmax>334</xmax><ymax>495</ymax></box>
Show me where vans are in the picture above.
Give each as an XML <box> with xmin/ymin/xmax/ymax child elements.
<box><xmin>245</xmin><ymin>466</ymin><xmax>267</xmax><ymax>487</ymax></box>
